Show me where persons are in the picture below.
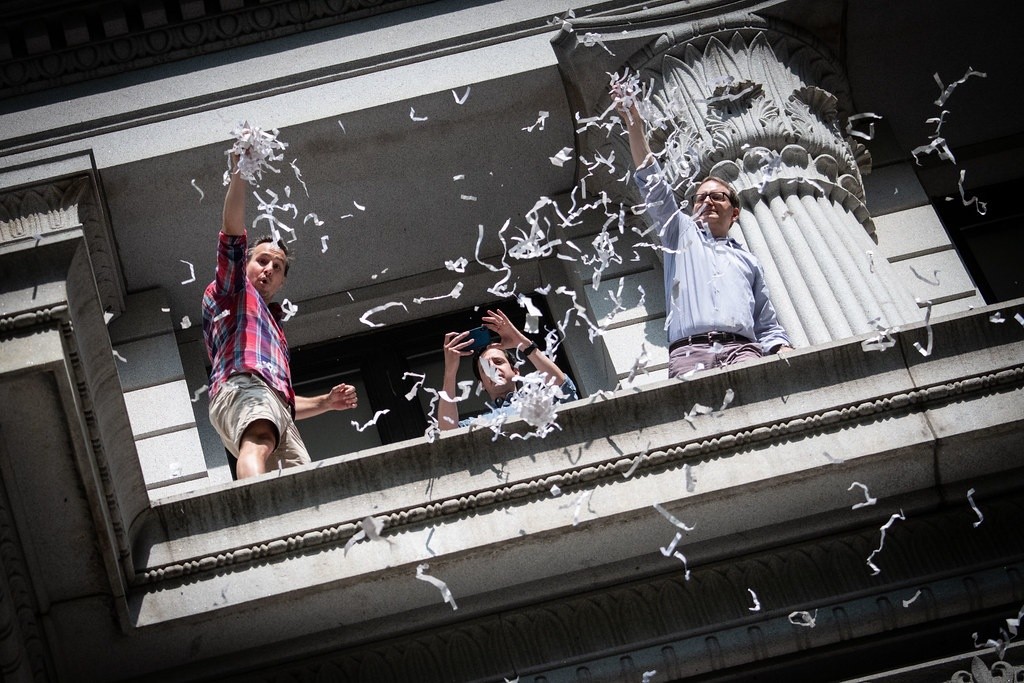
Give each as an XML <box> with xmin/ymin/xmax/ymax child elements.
<box><xmin>438</xmin><ymin>309</ymin><xmax>578</xmax><ymax>433</ymax></box>
<box><xmin>611</xmin><ymin>83</ymin><xmax>794</xmax><ymax>379</ymax></box>
<box><xmin>202</xmin><ymin>129</ymin><xmax>357</xmax><ymax>479</ymax></box>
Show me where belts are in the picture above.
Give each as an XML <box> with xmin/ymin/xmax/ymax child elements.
<box><xmin>669</xmin><ymin>332</ymin><xmax>752</xmax><ymax>353</ymax></box>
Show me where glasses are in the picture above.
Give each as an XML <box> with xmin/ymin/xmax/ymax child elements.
<box><xmin>692</xmin><ymin>192</ymin><xmax>735</xmax><ymax>209</ymax></box>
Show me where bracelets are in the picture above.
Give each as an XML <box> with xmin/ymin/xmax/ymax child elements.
<box><xmin>523</xmin><ymin>341</ymin><xmax>538</xmax><ymax>356</ymax></box>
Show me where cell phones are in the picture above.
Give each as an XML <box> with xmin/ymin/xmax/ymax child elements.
<box><xmin>450</xmin><ymin>326</ymin><xmax>491</xmax><ymax>352</ymax></box>
